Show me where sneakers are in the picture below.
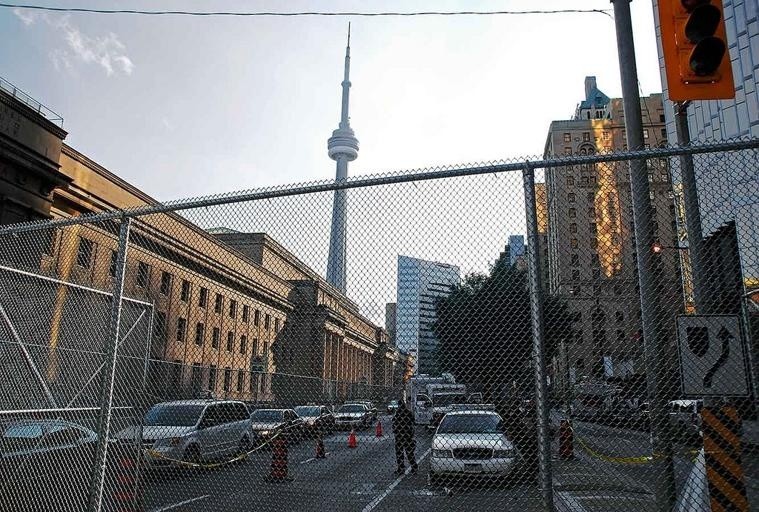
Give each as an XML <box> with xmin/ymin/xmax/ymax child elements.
<box><xmin>409</xmin><ymin>469</ymin><xmax>418</xmax><ymax>474</ymax></box>
<box><xmin>394</xmin><ymin>470</ymin><xmax>406</xmax><ymax>476</ymax></box>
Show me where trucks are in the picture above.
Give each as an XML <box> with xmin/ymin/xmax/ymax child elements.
<box><xmin>406</xmin><ymin>372</ymin><xmax>484</xmax><ymax>436</ymax></box>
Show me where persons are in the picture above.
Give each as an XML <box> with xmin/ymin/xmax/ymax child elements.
<box><xmin>392</xmin><ymin>399</ymin><xmax>418</xmax><ymax>475</ymax></box>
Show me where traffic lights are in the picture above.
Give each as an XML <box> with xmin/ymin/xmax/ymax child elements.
<box><xmin>657</xmin><ymin>0</ymin><xmax>736</xmax><ymax>101</ymax></box>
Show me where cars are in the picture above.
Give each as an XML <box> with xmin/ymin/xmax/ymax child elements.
<box><xmin>668</xmin><ymin>399</ymin><xmax>704</xmax><ymax>443</ymax></box>
<box><xmin>387</xmin><ymin>400</ymin><xmax>398</xmax><ymax>414</ymax></box>
<box><xmin>426</xmin><ymin>404</ymin><xmax>516</xmax><ymax>487</ymax></box>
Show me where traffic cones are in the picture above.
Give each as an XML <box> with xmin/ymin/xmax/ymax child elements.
<box><xmin>376</xmin><ymin>421</ymin><xmax>383</xmax><ymax>436</ymax></box>
